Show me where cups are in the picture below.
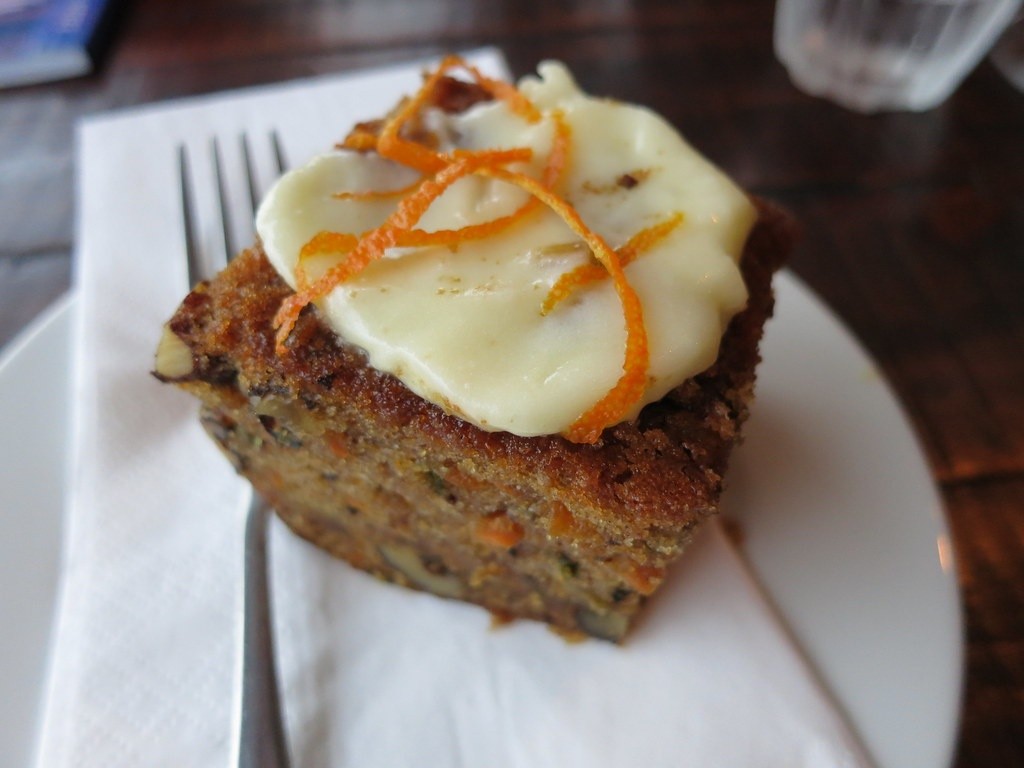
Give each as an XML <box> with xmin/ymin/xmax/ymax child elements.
<box><xmin>771</xmin><ymin>0</ymin><xmax>1024</xmax><ymax>115</ymax></box>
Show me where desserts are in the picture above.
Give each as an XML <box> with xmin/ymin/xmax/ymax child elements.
<box><xmin>154</xmin><ymin>52</ymin><xmax>779</xmax><ymax>640</ymax></box>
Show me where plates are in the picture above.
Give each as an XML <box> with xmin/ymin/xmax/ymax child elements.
<box><xmin>0</xmin><ymin>257</ymin><xmax>963</xmax><ymax>768</ymax></box>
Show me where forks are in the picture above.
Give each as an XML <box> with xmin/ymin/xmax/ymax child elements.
<box><xmin>168</xmin><ymin>125</ymin><xmax>300</xmax><ymax>768</ymax></box>
